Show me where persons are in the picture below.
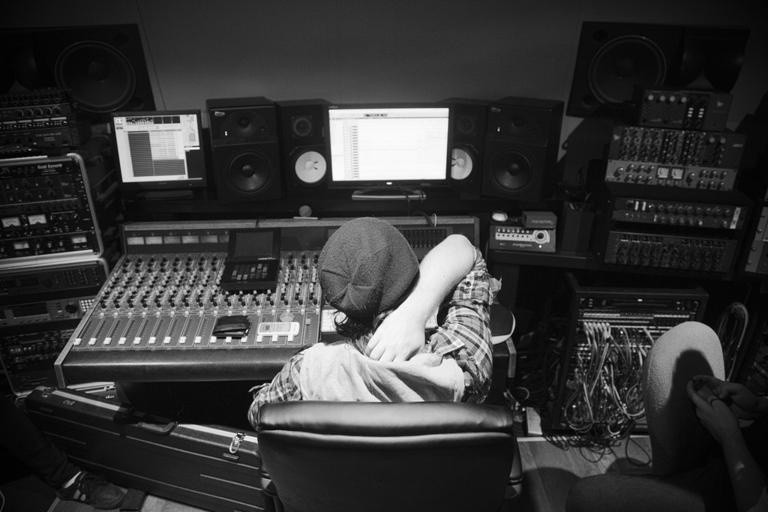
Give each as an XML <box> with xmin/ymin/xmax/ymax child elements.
<box><xmin>247</xmin><ymin>215</ymin><xmax>503</xmax><ymax>432</ymax></box>
<box><xmin>565</xmin><ymin>373</ymin><xmax>767</xmax><ymax>512</ymax></box>
<box><xmin>1</xmin><ymin>358</ymin><xmax>125</xmax><ymax>509</ymax></box>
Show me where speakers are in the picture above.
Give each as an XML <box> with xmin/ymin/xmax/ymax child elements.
<box><xmin>1</xmin><ymin>22</ymin><xmax>156</xmax><ymax>123</ymax></box>
<box><xmin>565</xmin><ymin>20</ymin><xmax>750</xmax><ymax>123</ymax></box>
<box><xmin>275</xmin><ymin>98</ymin><xmax>329</xmax><ymax>192</ymax></box>
<box><xmin>205</xmin><ymin>97</ymin><xmax>281</xmax><ymax>202</ymax></box>
<box><xmin>482</xmin><ymin>96</ymin><xmax>565</xmax><ymax>202</ymax></box>
<box><xmin>437</xmin><ymin>98</ymin><xmax>487</xmax><ymax>202</ymax></box>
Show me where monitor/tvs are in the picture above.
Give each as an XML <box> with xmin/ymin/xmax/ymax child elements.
<box><xmin>110</xmin><ymin>108</ymin><xmax>209</xmax><ymax>200</ymax></box>
<box><xmin>323</xmin><ymin>103</ymin><xmax>454</xmax><ymax>200</ymax></box>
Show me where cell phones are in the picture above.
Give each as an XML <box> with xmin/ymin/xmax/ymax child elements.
<box><xmin>693</xmin><ymin>380</ymin><xmax>731</xmax><ymax>406</ymax></box>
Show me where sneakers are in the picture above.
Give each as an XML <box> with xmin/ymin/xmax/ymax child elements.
<box><xmin>56</xmin><ymin>469</ymin><xmax>125</xmax><ymax>509</ymax></box>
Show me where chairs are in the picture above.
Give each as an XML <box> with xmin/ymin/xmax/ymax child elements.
<box><xmin>640</xmin><ymin>319</ymin><xmax>728</xmax><ymax>478</ymax></box>
<box><xmin>256</xmin><ymin>399</ymin><xmax>523</xmax><ymax>510</ymax></box>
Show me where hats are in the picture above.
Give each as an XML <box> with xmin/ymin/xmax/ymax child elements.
<box><xmin>317</xmin><ymin>217</ymin><xmax>420</xmax><ymax>318</ymax></box>
